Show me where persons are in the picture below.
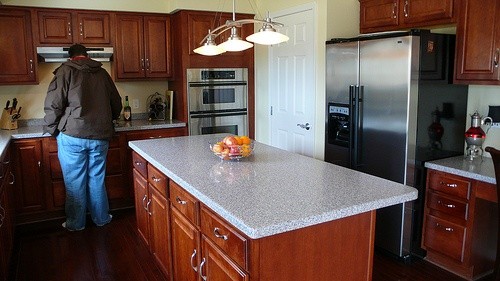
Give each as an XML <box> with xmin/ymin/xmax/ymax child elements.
<box><xmin>43</xmin><ymin>44</ymin><xmax>123</xmax><ymax>231</ymax></box>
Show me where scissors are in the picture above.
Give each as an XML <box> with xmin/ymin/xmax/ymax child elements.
<box><xmin>12</xmin><ymin>114</ymin><xmax>21</xmax><ymax>119</ymax></box>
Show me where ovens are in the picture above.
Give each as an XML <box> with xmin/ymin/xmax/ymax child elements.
<box><xmin>186</xmin><ymin>67</ymin><xmax>250</xmax><ymax>139</ymax></box>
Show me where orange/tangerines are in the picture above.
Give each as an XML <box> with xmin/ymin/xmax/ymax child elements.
<box><xmin>233</xmin><ymin>137</ymin><xmax>243</xmax><ymax>145</ymax></box>
<box><xmin>241</xmin><ymin>136</ymin><xmax>250</xmax><ymax>145</ymax></box>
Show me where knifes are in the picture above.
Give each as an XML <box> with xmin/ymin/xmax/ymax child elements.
<box><xmin>5</xmin><ymin>98</ymin><xmax>22</xmax><ymax>115</ymax></box>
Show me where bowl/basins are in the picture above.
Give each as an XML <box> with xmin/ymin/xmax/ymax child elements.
<box><xmin>208</xmin><ymin>135</ymin><xmax>256</xmax><ymax>160</ymax></box>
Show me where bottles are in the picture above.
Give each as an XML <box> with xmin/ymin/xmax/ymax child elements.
<box><xmin>123</xmin><ymin>96</ymin><xmax>131</xmax><ymax>122</ymax></box>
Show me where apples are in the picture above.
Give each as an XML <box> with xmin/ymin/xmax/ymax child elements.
<box><xmin>214</xmin><ymin>136</ymin><xmax>251</xmax><ymax>160</ymax></box>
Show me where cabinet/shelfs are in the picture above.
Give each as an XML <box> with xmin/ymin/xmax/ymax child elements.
<box><xmin>0</xmin><ymin>5</ymin><xmax>255</xmax><ymax>86</ymax></box>
<box><xmin>419</xmin><ymin>169</ymin><xmax>500</xmax><ymax>281</ymax></box>
<box><xmin>0</xmin><ymin>126</ymin><xmax>252</xmax><ymax>281</ymax></box>
<box><xmin>358</xmin><ymin>0</ymin><xmax>500</xmax><ymax>85</ymax></box>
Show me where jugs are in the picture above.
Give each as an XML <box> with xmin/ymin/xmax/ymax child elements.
<box><xmin>465</xmin><ymin>110</ymin><xmax>493</xmax><ymax>161</ymax></box>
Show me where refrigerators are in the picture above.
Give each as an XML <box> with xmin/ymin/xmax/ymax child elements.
<box><xmin>325</xmin><ymin>27</ymin><xmax>468</xmax><ymax>260</ymax></box>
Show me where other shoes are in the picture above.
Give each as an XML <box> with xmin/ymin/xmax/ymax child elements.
<box><xmin>62</xmin><ymin>222</ymin><xmax>84</xmax><ymax>231</ymax></box>
<box><xmin>97</xmin><ymin>214</ymin><xmax>112</xmax><ymax>226</ymax></box>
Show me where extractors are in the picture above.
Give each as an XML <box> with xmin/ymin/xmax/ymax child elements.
<box><xmin>36</xmin><ymin>46</ymin><xmax>114</xmax><ymax>63</ymax></box>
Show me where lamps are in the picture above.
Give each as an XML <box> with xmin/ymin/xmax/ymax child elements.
<box><xmin>192</xmin><ymin>0</ymin><xmax>290</xmax><ymax>56</ymax></box>
